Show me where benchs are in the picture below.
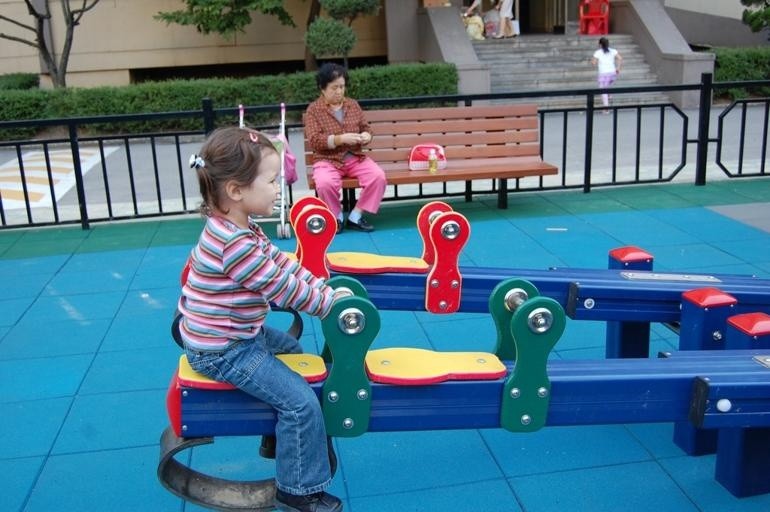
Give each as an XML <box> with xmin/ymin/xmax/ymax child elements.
<box><xmin>301</xmin><ymin>101</ymin><xmax>557</xmax><ymax>215</ymax></box>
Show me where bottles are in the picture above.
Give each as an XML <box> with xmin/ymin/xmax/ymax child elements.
<box><xmin>428</xmin><ymin>149</ymin><xmax>437</xmax><ymax>173</ymax></box>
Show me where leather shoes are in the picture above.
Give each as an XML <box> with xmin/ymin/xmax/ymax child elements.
<box><xmin>274</xmin><ymin>489</ymin><xmax>343</xmax><ymax>512</ymax></box>
<box><xmin>336</xmin><ymin>217</ymin><xmax>345</xmax><ymax>234</ymax></box>
<box><xmin>347</xmin><ymin>217</ymin><xmax>373</xmax><ymax>231</ymax></box>
<box><xmin>258</xmin><ymin>434</ymin><xmax>277</xmax><ymax>459</ymax></box>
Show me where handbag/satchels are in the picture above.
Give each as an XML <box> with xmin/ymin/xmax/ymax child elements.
<box><xmin>408</xmin><ymin>144</ymin><xmax>447</xmax><ymax>170</ymax></box>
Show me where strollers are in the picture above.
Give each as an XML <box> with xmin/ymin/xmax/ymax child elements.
<box><xmin>236</xmin><ymin>102</ymin><xmax>298</xmax><ymax>239</ymax></box>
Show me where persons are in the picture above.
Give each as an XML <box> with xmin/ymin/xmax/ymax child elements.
<box><xmin>177</xmin><ymin>128</ymin><xmax>352</xmax><ymax>511</ymax></box>
<box><xmin>305</xmin><ymin>62</ymin><xmax>388</xmax><ymax>234</ymax></box>
<box><xmin>464</xmin><ymin>0</ymin><xmax>516</xmax><ymax>39</ymax></box>
<box><xmin>591</xmin><ymin>37</ymin><xmax>623</xmax><ymax>115</ymax></box>
